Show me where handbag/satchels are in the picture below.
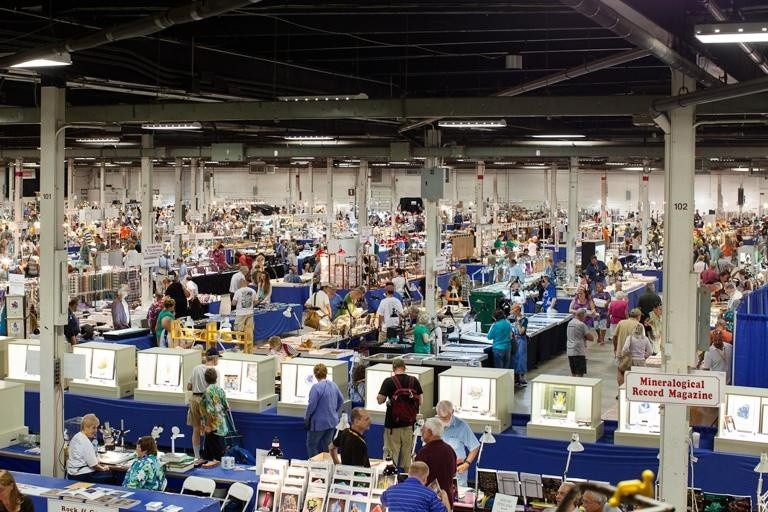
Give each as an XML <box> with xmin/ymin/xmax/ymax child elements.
<box><xmin>303</xmin><ymin>311</ymin><xmax>320</xmax><ymax>329</ymax></box>
<box><xmin>617</xmin><ymin>352</ymin><xmax>632</xmax><ymax>371</ymax></box>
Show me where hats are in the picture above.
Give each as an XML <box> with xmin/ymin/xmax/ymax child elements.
<box><xmin>321</xmin><ymin>281</ymin><xmax>332</xmax><ymax>287</ymax></box>
<box><xmin>205</xmin><ymin>348</ymin><xmax>222</xmax><ymax>357</ymax></box>
<box><xmin>629</xmin><ymin>309</ymin><xmax>644</xmax><ymax>317</ymax></box>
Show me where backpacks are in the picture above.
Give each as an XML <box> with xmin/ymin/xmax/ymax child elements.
<box><xmin>390</xmin><ymin>376</ymin><xmax>420</xmax><ymax>425</ymax></box>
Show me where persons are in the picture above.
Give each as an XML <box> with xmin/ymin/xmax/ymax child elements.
<box><xmin>0</xmin><ymin>194</ymin><xmax>767</xmax><ymax>512</ymax></box>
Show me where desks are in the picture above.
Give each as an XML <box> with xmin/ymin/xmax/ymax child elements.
<box><xmin>0</xmin><ymin>225</ymin><xmax>768</xmax><ymax>512</ymax></box>
